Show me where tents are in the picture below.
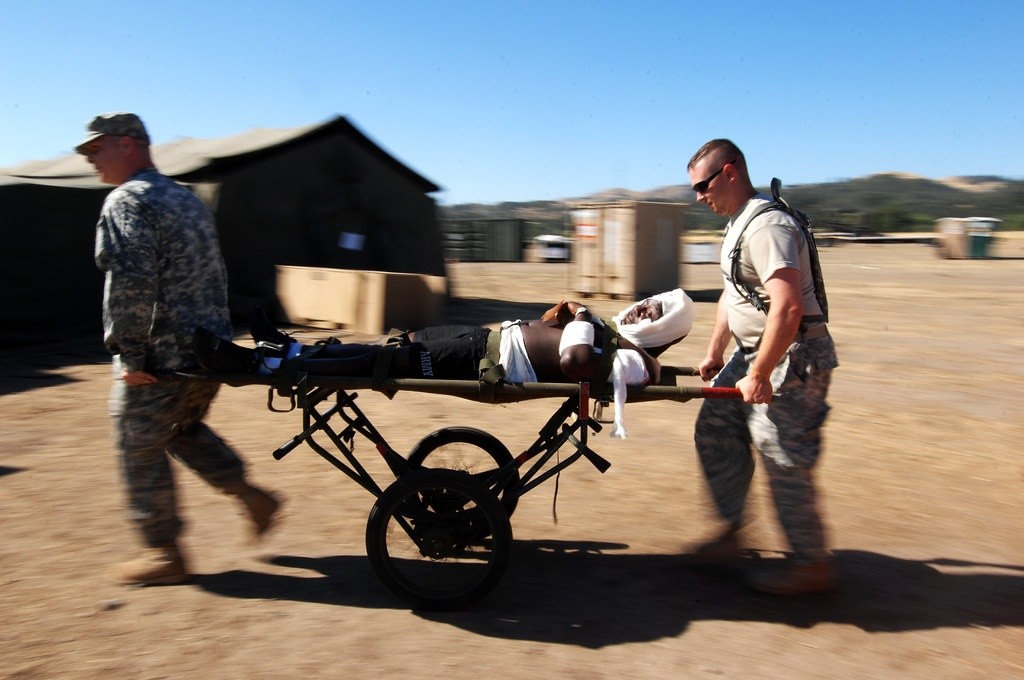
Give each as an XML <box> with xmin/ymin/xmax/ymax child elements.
<box><xmin>0</xmin><ymin>115</ymin><xmax>441</xmax><ymax>370</ymax></box>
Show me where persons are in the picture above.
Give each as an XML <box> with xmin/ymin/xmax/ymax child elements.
<box><xmin>685</xmin><ymin>139</ymin><xmax>838</xmax><ymax>594</ymax></box>
<box><xmin>74</xmin><ymin>112</ymin><xmax>283</xmax><ymax>587</ymax></box>
<box><xmin>190</xmin><ymin>287</ymin><xmax>696</xmax><ymax>388</ymax></box>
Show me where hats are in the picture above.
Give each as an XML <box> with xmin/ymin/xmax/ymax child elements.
<box><xmin>75</xmin><ymin>112</ymin><xmax>150</xmax><ymax>155</ymax></box>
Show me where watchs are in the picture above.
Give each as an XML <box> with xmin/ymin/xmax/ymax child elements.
<box><xmin>575</xmin><ymin>307</ymin><xmax>592</xmax><ymax>319</ymax></box>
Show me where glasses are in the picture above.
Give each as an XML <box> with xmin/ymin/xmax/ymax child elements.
<box><xmin>693</xmin><ymin>159</ymin><xmax>736</xmax><ymax>193</ymax></box>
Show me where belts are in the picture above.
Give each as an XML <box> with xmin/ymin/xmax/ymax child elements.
<box><xmin>739</xmin><ymin>323</ymin><xmax>828</xmax><ymax>354</ymax></box>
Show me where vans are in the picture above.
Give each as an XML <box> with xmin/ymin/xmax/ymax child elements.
<box><xmin>532</xmin><ymin>234</ymin><xmax>570</xmax><ymax>261</ymax></box>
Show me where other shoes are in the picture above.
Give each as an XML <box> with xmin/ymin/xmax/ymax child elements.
<box><xmin>110</xmin><ymin>544</ymin><xmax>186</xmax><ymax>586</ymax></box>
<box><xmin>684</xmin><ymin>527</ymin><xmax>746</xmax><ymax>561</ymax></box>
<box><xmin>238</xmin><ymin>487</ymin><xmax>278</xmax><ymax>538</ymax></box>
<box><xmin>194</xmin><ymin>327</ymin><xmax>245</xmax><ymax>376</ymax></box>
<box><xmin>250</xmin><ymin>309</ymin><xmax>291</xmax><ymax>344</ymax></box>
<box><xmin>753</xmin><ymin>559</ymin><xmax>831</xmax><ymax>595</ymax></box>
